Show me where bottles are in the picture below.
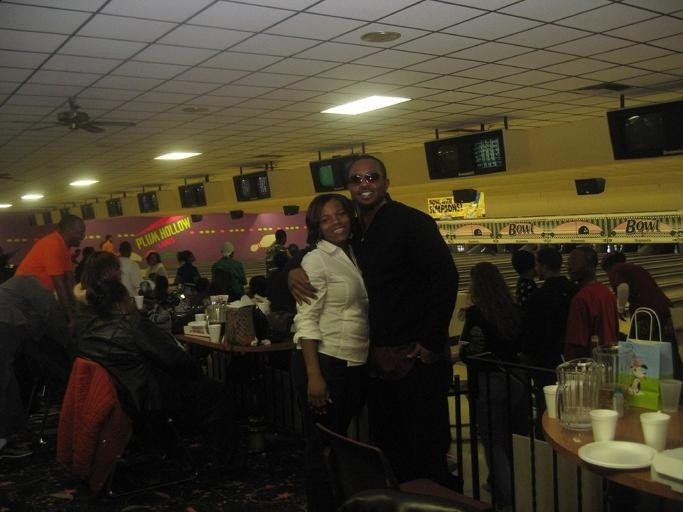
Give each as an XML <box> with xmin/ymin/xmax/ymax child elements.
<box><xmin>612</xmin><ymin>387</ymin><xmax>624</xmax><ymax>420</ymax></box>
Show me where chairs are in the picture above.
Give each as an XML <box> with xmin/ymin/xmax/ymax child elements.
<box><xmin>52</xmin><ymin>337</ymin><xmax>203</xmax><ymax>509</ymax></box>
<box><xmin>11</xmin><ymin>333</ymin><xmax>75</xmax><ymax>450</ymax></box>
<box><xmin>311</xmin><ymin>419</ymin><xmax>500</xmax><ymax>512</ymax></box>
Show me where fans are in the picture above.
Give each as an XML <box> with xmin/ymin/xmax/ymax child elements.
<box><xmin>6</xmin><ymin>94</ymin><xmax>137</xmax><ymax>138</ymax></box>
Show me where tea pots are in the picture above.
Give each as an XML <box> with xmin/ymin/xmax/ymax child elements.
<box><xmin>554</xmin><ymin>357</ymin><xmax>606</xmax><ymax>431</ymax></box>
<box><xmin>204</xmin><ymin>295</ymin><xmax>228</xmax><ymax>336</ymax></box>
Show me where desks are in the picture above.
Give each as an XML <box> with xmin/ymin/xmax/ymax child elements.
<box><xmin>167</xmin><ymin>322</ymin><xmax>307</xmax><ymax>484</ymax></box>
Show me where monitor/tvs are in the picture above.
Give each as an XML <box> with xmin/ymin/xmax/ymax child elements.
<box><xmin>61</xmin><ymin>208</ymin><xmax>70</xmax><ymax>217</ymax></box>
<box><xmin>27</xmin><ymin>215</ymin><xmax>36</xmax><ymax>226</ymax></box>
<box><xmin>424</xmin><ymin>130</ymin><xmax>506</xmax><ymax>179</ymax></box>
<box><xmin>43</xmin><ymin>212</ymin><xmax>52</xmax><ymax>225</ymax></box>
<box><xmin>107</xmin><ymin>198</ymin><xmax>122</xmax><ymax>216</ymax></box>
<box><xmin>178</xmin><ymin>183</ymin><xmax>206</xmax><ymax>208</ymax></box>
<box><xmin>138</xmin><ymin>191</ymin><xmax>158</xmax><ymax>212</ymax></box>
<box><xmin>233</xmin><ymin>171</ymin><xmax>271</xmax><ymax>202</ymax></box>
<box><xmin>81</xmin><ymin>204</ymin><xmax>95</xmax><ymax>219</ymax></box>
<box><xmin>309</xmin><ymin>155</ymin><xmax>359</xmax><ymax>193</ymax></box>
<box><xmin>607</xmin><ymin>100</ymin><xmax>683</xmax><ymax>161</ymax></box>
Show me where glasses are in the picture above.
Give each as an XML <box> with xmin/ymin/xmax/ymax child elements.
<box><xmin>349</xmin><ymin>171</ymin><xmax>380</xmax><ymax>185</ymax></box>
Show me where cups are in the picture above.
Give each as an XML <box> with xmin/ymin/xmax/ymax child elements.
<box><xmin>207</xmin><ymin>324</ymin><xmax>221</xmax><ymax>345</ymax></box>
<box><xmin>134</xmin><ymin>296</ymin><xmax>144</xmax><ymax>310</ymax></box>
<box><xmin>659</xmin><ymin>379</ymin><xmax>682</xmax><ymax>415</ymax></box>
<box><xmin>544</xmin><ymin>384</ymin><xmax>565</xmax><ymax>419</ymax></box>
<box><xmin>195</xmin><ymin>314</ymin><xmax>204</xmax><ymax>322</ymax></box>
<box><xmin>589</xmin><ymin>408</ymin><xmax>620</xmax><ymax>442</ymax></box>
<box><xmin>592</xmin><ymin>340</ymin><xmax>633</xmax><ymax>415</ymax></box>
<box><xmin>639</xmin><ymin>411</ymin><xmax>671</xmax><ymax>450</ymax></box>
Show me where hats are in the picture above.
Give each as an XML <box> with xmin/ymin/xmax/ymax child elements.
<box><xmin>223</xmin><ymin>242</ymin><xmax>234</xmax><ymax>256</ymax></box>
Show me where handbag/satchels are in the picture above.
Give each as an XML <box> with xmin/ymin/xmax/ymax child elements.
<box><xmin>226</xmin><ymin>306</ymin><xmax>272</xmax><ymax>346</ymax></box>
<box><xmin>618</xmin><ymin>338</ymin><xmax>674</xmax><ymax>410</ymax></box>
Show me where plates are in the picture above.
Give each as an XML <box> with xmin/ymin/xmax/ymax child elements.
<box><xmin>654</xmin><ymin>447</ymin><xmax>683</xmax><ymax>482</ymax></box>
<box><xmin>186</xmin><ymin>321</ymin><xmax>206</xmax><ymax>327</ymax></box>
<box><xmin>574</xmin><ymin>441</ymin><xmax>657</xmax><ymax>472</ymax></box>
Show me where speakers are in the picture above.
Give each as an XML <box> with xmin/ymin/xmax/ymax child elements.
<box><xmin>191</xmin><ymin>215</ymin><xmax>203</xmax><ymax>222</ymax></box>
<box><xmin>453</xmin><ymin>189</ymin><xmax>476</xmax><ymax>202</ymax></box>
<box><xmin>231</xmin><ymin>210</ymin><xmax>243</xmax><ymax>219</ymax></box>
<box><xmin>575</xmin><ymin>178</ymin><xmax>606</xmax><ymax>195</ymax></box>
<box><xmin>283</xmin><ymin>206</ymin><xmax>299</xmax><ymax>216</ymax></box>
